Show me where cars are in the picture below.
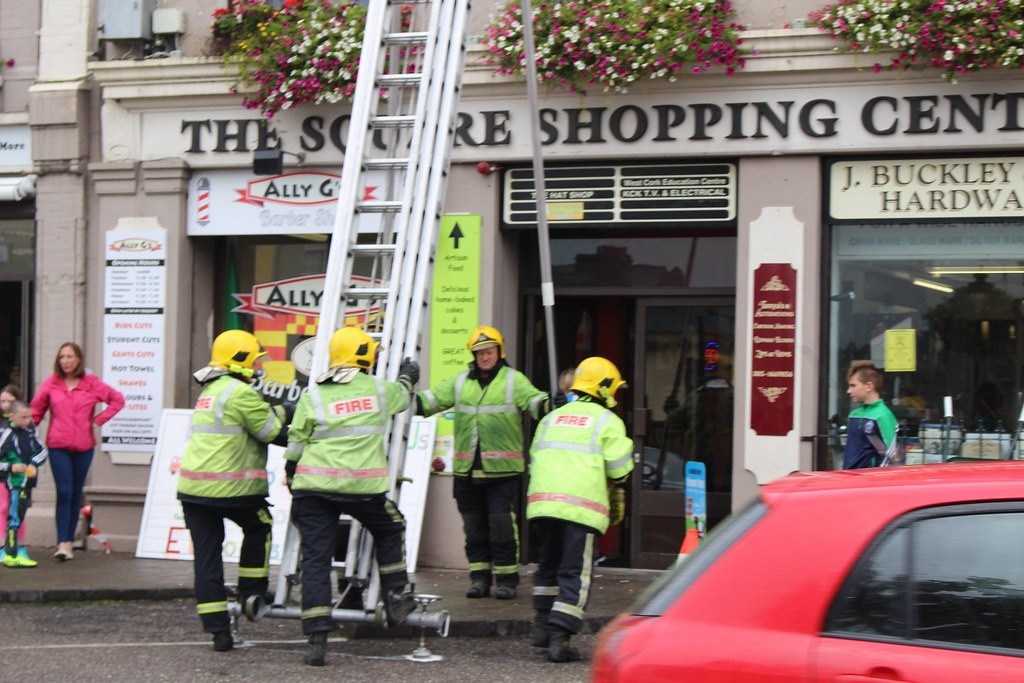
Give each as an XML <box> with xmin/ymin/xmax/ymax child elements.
<box><xmin>592</xmin><ymin>460</ymin><xmax>1024</xmax><ymax>683</ymax></box>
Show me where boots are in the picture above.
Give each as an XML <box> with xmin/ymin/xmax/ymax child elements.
<box><xmin>549</xmin><ymin>626</ymin><xmax>580</xmax><ymax>662</ymax></box>
<box><xmin>384</xmin><ymin>589</ymin><xmax>417</xmax><ymax>627</ymax></box>
<box><xmin>304</xmin><ymin>632</ymin><xmax>326</xmax><ymax>666</ymax></box>
<box><xmin>534</xmin><ymin>610</ymin><xmax>549</xmax><ymax>646</ymax></box>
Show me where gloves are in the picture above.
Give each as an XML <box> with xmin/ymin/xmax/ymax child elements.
<box><xmin>549</xmin><ymin>388</ymin><xmax>567</xmax><ymax>408</ymax></box>
<box><xmin>401</xmin><ymin>357</ymin><xmax>419</xmax><ymax>385</ymax></box>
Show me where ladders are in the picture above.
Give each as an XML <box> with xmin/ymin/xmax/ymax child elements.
<box><xmin>272</xmin><ymin>0</ymin><xmax>469</xmax><ymax>605</ymax></box>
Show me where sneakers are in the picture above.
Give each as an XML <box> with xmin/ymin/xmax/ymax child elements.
<box><xmin>4</xmin><ymin>552</ymin><xmax>37</xmax><ymax>568</ymax></box>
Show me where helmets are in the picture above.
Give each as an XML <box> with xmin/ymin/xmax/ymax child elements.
<box><xmin>569</xmin><ymin>356</ymin><xmax>626</xmax><ymax>406</ymax></box>
<box><xmin>208</xmin><ymin>329</ymin><xmax>268</xmax><ymax>369</ymax></box>
<box><xmin>327</xmin><ymin>327</ymin><xmax>380</xmax><ymax>368</ymax></box>
<box><xmin>468</xmin><ymin>326</ymin><xmax>506</xmax><ymax>359</ymax></box>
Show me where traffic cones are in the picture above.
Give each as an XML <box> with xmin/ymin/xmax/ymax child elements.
<box><xmin>676</xmin><ymin>530</ymin><xmax>699</xmax><ymax>559</ymax></box>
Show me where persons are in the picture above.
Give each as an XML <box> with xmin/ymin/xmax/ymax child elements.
<box><xmin>948</xmin><ymin>441</ymin><xmax>956</xmax><ymax>455</ymax></box>
<box><xmin>29</xmin><ymin>343</ymin><xmax>125</xmax><ymax>561</ymax></box>
<box><xmin>409</xmin><ymin>325</ymin><xmax>569</xmax><ymax>599</ymax></box>
<box><xmin>928</xmin><ymin>442</ymin><xmax>938</xmax><ymax>454</ymax></box>
<box><xmin>843</xmin><ymin>361</ymin><xmax>900</xmax><ymax>470</ymax></box>
<box><xmin>282</xmin><ymin>327</ymin><xmax>421</xmax><ymax>666</ymax></box>
<box><xmin>682</xmin><ymin>354</ymin><xmax>734</xmax><ymax>535</ymax></box>
<box><xmin>0</xmin><ymin>384</ymin><xmax>48</xmax><ymax>567</ymax></box>
<box><xmin>525</xmin><ymin>356</ymin><xmax>634</xmax><ymax>663</ymax></box>
<box><xmin>176</xmin><ymin>329</ymin><xmax>298</xmax><ymax>652</ymax></box>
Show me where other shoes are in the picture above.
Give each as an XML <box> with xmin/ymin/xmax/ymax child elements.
<box><xmin>238</xmin><ymin>588</ymin><xmax>275</xmax><ymax>604</ymax></box>
<box><xmin>56</xmin><ymin>550</ymin><xmax>74</xmax><ymax>560</ymax></box>
<box><xmin>495</xmin><ymin>585</ymin><xmax>517</xmax><ymax>598</ymax></box>
<box><xmin>213</xmin><ymin>632</ymin><xmax>234</xmax><ymax>650</ymax></box>
<box><xmin>467</xmin><ymin>582</ymin><xmax>490</xmax><ymax>597</ymax></box>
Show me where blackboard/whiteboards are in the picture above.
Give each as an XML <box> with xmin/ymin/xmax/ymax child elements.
<box><xmin>135</xmin><ymin>407</ymin><xmax>438</xmax><ymax>584</ymax></box>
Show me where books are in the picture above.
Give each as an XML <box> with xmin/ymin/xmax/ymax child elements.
<box><xmin>905</xmin><ymin>427</ymin><xmax>1024</xmax><ymax>465</ymax></box>
